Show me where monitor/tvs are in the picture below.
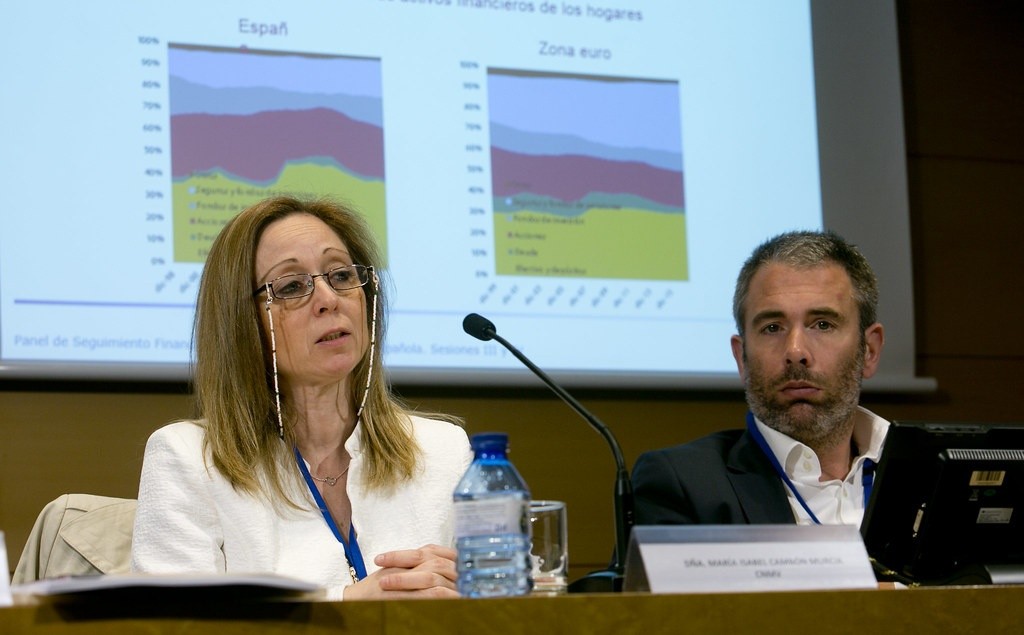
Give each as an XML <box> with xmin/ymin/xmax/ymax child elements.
<box><xmin>860</xmin><ymin>419</ymin><xmax>1023</xmax><ymax>584</ymax></box>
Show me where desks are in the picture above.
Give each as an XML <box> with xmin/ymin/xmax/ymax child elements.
<box><xmin>0</xmin><ymin>584</ymin><xmax>1024</xmax><ymax>635</ymax></box>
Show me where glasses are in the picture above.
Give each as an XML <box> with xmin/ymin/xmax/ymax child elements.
<box><xmin>249</xmin><ymin>262</ymin><xmax>373</xmax><ymax>300</ymax></box>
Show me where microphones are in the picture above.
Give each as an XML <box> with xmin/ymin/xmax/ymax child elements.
<box><xmin>463</xmin><ymin>314</ymin><xmax>643</xmax><ymax>596</ymax></box>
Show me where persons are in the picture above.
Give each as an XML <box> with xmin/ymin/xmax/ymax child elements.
<box><xmin>121</xmin><ymin>194</ymin><xmax>478</xmax><ymax>597</ymax></box>
<box><xmin>625</xmin><ymin>230</ymin><xmax>992</xmax><ymax>586</ymax></box>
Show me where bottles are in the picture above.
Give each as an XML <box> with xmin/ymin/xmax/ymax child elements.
<box><xmin>453</xmin><ymin>434</ymin><xmax>534</xmax><ymax>599</ymax></box>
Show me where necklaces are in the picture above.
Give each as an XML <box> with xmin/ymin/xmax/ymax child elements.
<box><xmin>308</xmin><ymin>454</ymin><xmax>351</xmax><ymax>487</ymax></box>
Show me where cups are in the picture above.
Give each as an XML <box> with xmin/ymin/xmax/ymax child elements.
<box><xmin>525</xmin><ymin>500</ymin><xmax>569</xmax><ymax>598</ymax></box>
